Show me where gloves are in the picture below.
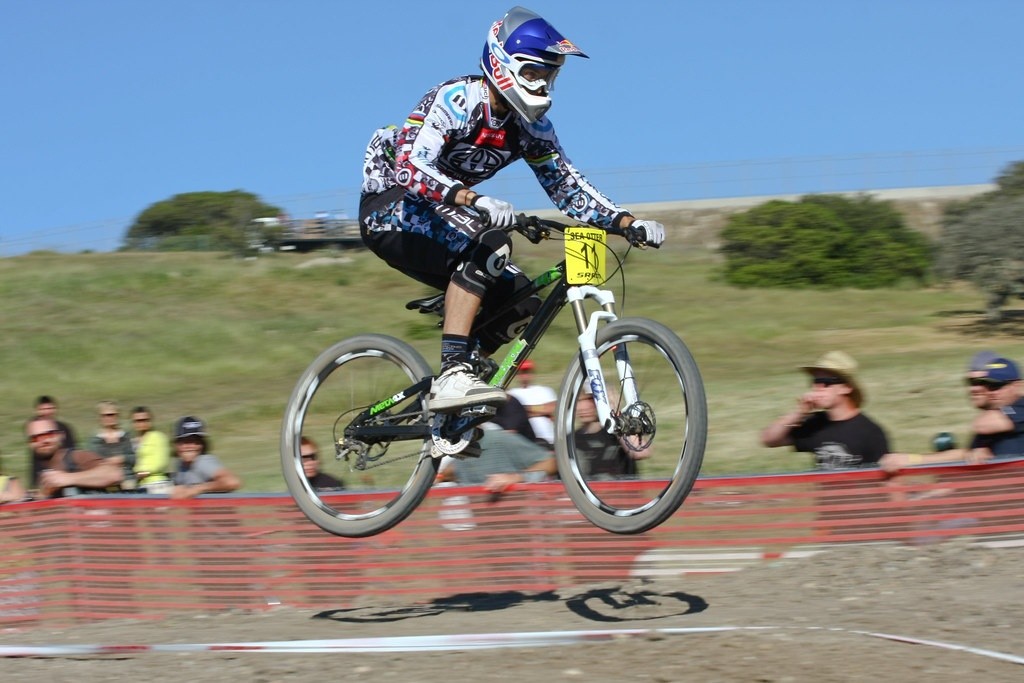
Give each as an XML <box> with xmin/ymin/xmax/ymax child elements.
<box><xmin>469</xmin><ymin>194</ymin><xmax>517</xmax><ymax>230</ymax></box>
<box><xmin>624</xmin><ymin>220</ymin><xmax>664</xmax><ymax>250</ymax></box>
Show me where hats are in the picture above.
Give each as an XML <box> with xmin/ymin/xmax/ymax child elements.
<box><xmin>172</xmin><ymin>417</ymin><xmax>211</xmax><ymax>438</ymax></box>
<box><xmin>973</xmin><ymin>358</ymin><xmax>1020</xmax><ymax>381</ymax></box>
<box><xmin>799</xmin><ymin>351</ymin><xmax>863</xmax><ymax>408</ymax></box>
<box><xmin>519</xmin><ymin>361</ymin><xmax>535</xmax><ymax>370</ymax></box>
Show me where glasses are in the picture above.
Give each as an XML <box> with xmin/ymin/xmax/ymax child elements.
<box><xmin>28</xmin><ymin>429</ymin><xmax>65</xmax><ymax>443</ymax></box>
<box><xmin>967</xmin><ymin>379</ymin><xmax>1022</xmax><ymax>390</ymax></box>
<box><xmin>814</xmin><ymin>377</ymin><xmax>844</xmax><ymax>385</ymax></box>
<box><xmin>514</xmin><ymin>61</ymin><xmax>560</xmax><ymax>93</ymax></box>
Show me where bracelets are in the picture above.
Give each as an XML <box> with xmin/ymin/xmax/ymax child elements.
<box><xmin>465</xmin><ymin>191</ymin><xmax>476</xmax><ymax>207</ymax></box>
<box><xmin>438</xmin><ymin>474</ymin><xmax>443</xmax><ymax>477</ymax></box>
<box><xmin>908</xmin><ymin>454</ymin><xmax>920</xmax><ymax>464</ymax></box>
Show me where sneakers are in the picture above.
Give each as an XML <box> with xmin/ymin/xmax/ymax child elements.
<box><xmin>429</xmin><ymin>363</ymin><xmax>508</xmax><ymax>415</ymax></box>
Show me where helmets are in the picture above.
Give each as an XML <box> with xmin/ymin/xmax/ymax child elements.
<box><xmin>479</xmin><ymin>6</ymin><xmax>590</xmax><ymax>124</ymax></box>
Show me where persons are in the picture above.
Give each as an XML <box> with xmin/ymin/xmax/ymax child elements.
<box><xmin>0</xmin><ymin>476</ymin><xmax>44</xmax><ymax>615</ymax></box>
<box><xmin>760</xmin><ymin>349</ymin><xmax>889</xmax><ymax>544</ymax></box>
<box><xmin>22</xmin><ymin>395</ymin><xmax>250</xmax><ymax>619</ymax></box>
<box><xmin>300</xmin><ymin>438</ymin><xmax>364</xmax><ymax>606</ymax></box>
<box><xmin>435</xmin><ymin>358</ymin><xmax>651</xmax><ymax>585</ymax></box>
<box><xmin>360</xmin><ymin>7</ymin><xmax>666</xmax><ymax>412</ymax></box>
<box><xmin>878</xmin><ymin>349</ymin><xmax>1024</xmax><ymax>543</ymax></box>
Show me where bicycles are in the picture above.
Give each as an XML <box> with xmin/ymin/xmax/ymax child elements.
<box><xmin>276</xmin><ymin>211</ymin><xmax>711</xmax><ymax>540</ymax></box>
